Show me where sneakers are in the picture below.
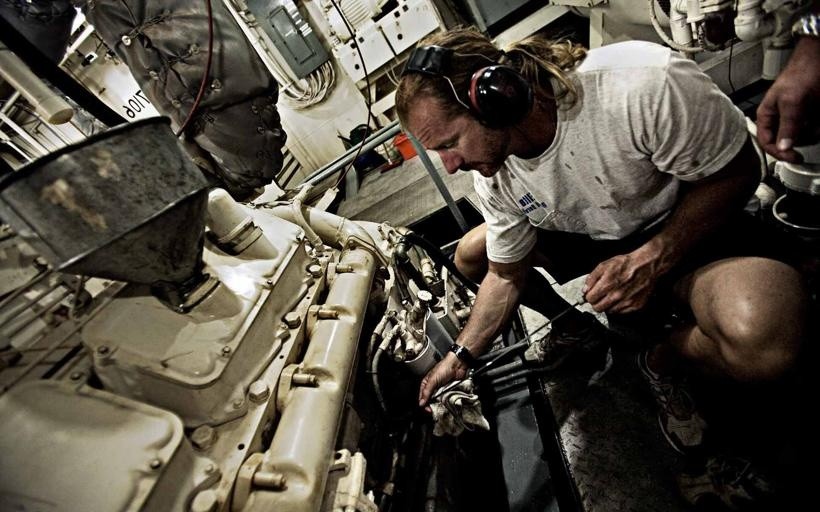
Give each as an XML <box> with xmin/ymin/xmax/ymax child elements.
<box><xmin>523</xmin><ymin>312</ymin><xmax>609</xmax><ymax>370</ymax></box>
<box><xmin>637</xmin><ymin>344</ymin><xmax>707</xmax><ymax>452</ymax></box>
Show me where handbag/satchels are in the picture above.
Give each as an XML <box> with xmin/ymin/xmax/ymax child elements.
<box><xmin>337</xmin><ymin>123</ymin><xmax>375</xmax><ymax>175</ymax></box>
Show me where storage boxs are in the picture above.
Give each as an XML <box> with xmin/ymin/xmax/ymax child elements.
<box><xmin>392</xmin><ymin>134</ymin><xmax>417</xmax><ymax>161</ymax></box>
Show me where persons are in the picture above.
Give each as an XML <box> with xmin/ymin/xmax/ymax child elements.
<box><xmin>755</xmin><ymin>8</ymin><xmax>820</xmax><ymax>167</ymax></box>
<box><xmin>394</xmin><ymin>24</ymin><xmax>806</xmax><ymax>456</ymax></box>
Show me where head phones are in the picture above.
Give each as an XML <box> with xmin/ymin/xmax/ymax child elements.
<box><xmin>397</xmin><ymin>43</ymin><xmax>535</xmax><ymax>129</ymax></box>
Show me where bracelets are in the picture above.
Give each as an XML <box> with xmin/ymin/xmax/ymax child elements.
<box><xmin>447</xmin><ymin>344</ymin><xmax>474</xmax><ymax>369</ymax></box>
<box><xmin>791</xmin><ymin>14</ymin><xmax>820</xmax><ymax>37</ymax></box>
